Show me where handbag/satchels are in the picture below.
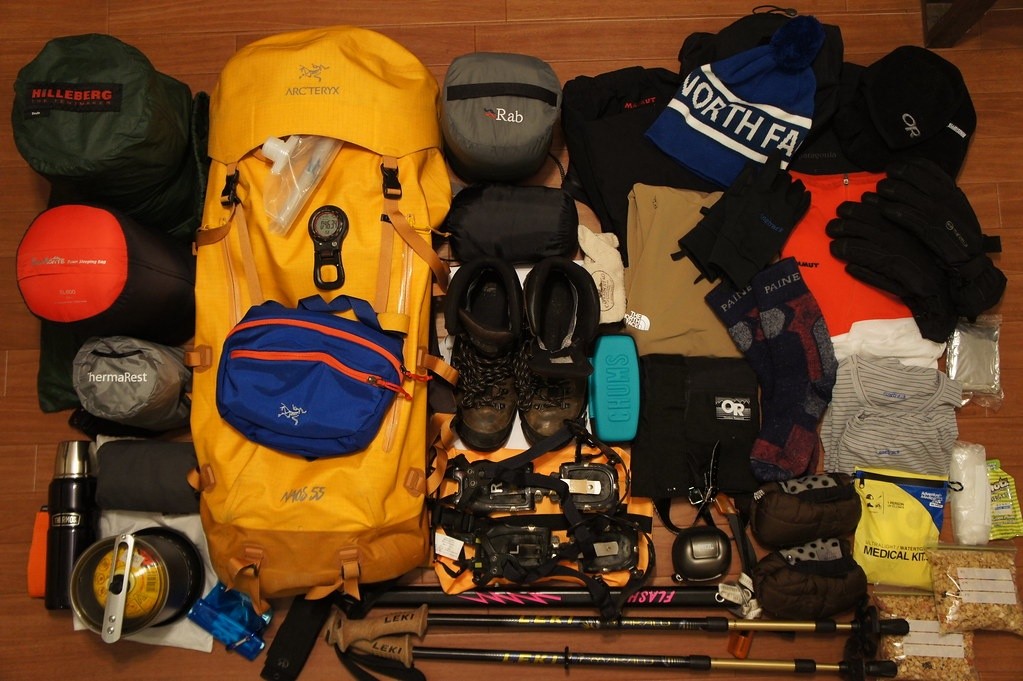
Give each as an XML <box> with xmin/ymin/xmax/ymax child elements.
<box><xmin>215</xmin><ymin>294</ymin><xmax>406</xmax><ymax>458</ymax></box>
<box><xmin>852</xmin><ymin>466</ymin><xmax>964</xmax><ymax>591</ymax></box>
<box><xmin>560</xmin><ymin>65</ymin><xmax>679</xmax><ymax>266</ymax></box>
<box><xmin>630</xmin><ymin>354</ymin><xmax>757</xmax><ymax>539</ymax></box>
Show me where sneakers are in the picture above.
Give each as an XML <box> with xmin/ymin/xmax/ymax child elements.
<box><xmin>441</xmin><ymin>257</ymin><xmax>517</xmax><ymax>452</ymax></box>
<box><xmin>515</xmin><ymin>259</ymin><xmax>601</xmax><ymax>447</ymax></box>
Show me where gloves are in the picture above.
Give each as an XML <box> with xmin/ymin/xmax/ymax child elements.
<box><xmin>862</xmin><ymin>162</ymin><xmax>1007</xmax><ymax>322</ymax></box>
<box><xmin>670</xmin><ymin>162</ymin><xmax>812</xmax><ymax>286</ymax></box>
<box><xmin>825</xmin><ymin>193</ymin><xmax>961</xmax><ymax>343</ymax></box>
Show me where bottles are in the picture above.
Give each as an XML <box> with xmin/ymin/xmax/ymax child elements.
<box><xmin>45</xmin><ymin>440</ymin><xmax>97</xmax><ymax>611</ymax></box>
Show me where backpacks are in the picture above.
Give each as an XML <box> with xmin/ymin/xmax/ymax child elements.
<box><xmin>182</xmin><ymin>25</ymin><xmax>458</xmax><ymax>617</ymax></box>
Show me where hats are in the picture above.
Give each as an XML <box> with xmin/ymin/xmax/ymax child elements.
<box><xmin>864</xmin><ymin>44</ymin><xmax>976</xmax><ymax>178</ymax></box>
